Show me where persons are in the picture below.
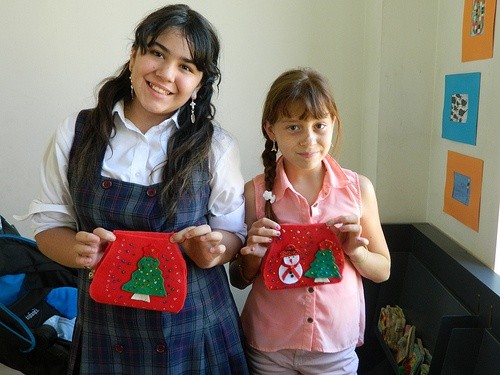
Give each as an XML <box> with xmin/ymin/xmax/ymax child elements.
<box><xmin>12</xmin><ymin>2</ymin><xmax>247</xmax><ymax>375</ymax></box>
<box><xmin>228</xmin><ymin>69</ymin><xmax>393</xmax><ymax>374</ymax></box>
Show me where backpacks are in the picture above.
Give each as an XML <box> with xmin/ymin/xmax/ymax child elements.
<box><xmin>0</xmin><ymin>215</ymin><xmax>80</xmax><ymax>375</ymax></box>
